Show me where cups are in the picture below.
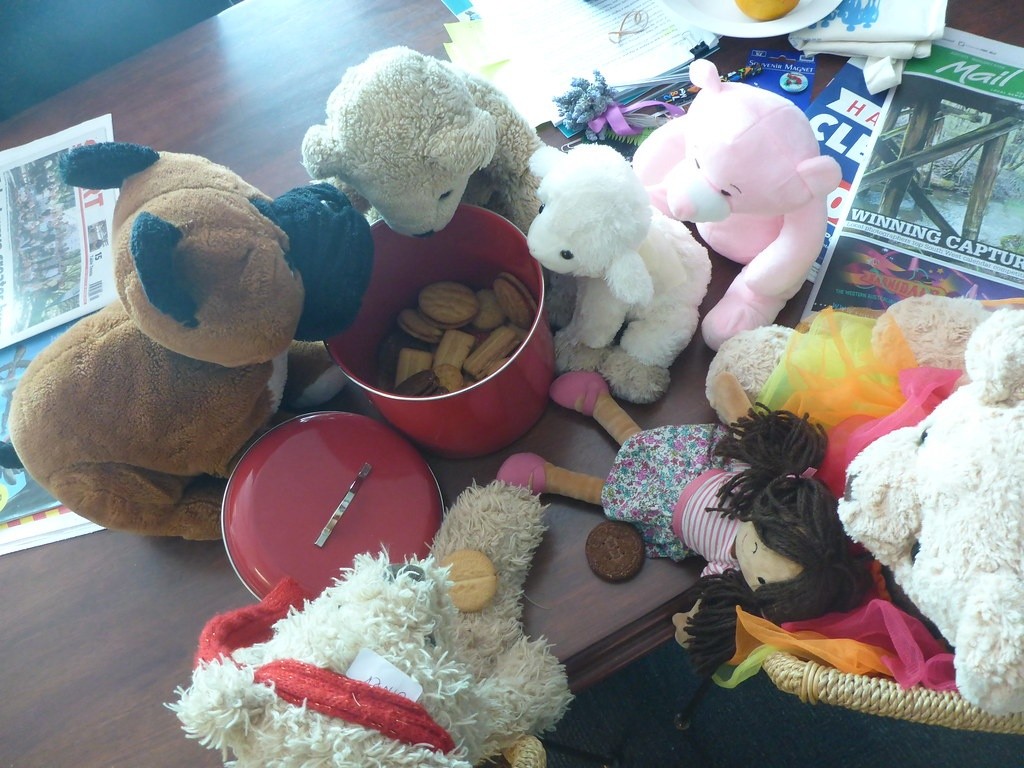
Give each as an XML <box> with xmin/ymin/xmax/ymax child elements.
<box><xmin>323</xmin><ymin>205</ymin><xmax>557</xmax><ymax>457</ymax></box>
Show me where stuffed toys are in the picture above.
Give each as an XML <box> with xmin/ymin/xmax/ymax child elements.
<box><xmin>702</xmin><ymin>295</ymin><xmax>1023</xmax><ymax>716</ymax></box>
<box><xmin>524</xmin><ymin>141</ymin><xmax>713</xmax><ymax>407</ymax></box>
<box><xmin>5</xmin><ymin>141</ymin><xmax>378</xmax><ymax>546</ymax></box>
<box><xmin>498</xmin><ymin>370</ymin><xmax>874</xmax><ymax>718</ymax></box>
<box><xmin>158</xmin><ymin>481</ymin><xmax>578</xmax><ymax>768</ymax></box>
<box><xmin>632</xmin><ymin>56</ymin><xmax>846</xmax><ymax>355</ymax></box>
<box><xmin>302</xmin><ymin>43</ymin><xmax>543</xmax><ymax>244</ymax></box>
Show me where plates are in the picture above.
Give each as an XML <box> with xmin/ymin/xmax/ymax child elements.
<box><xmin>649</xmin><ymin>0</ymin><xmax>845</xmax><ymax>37</ymax></box>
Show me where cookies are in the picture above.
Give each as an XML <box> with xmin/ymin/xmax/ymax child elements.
<box><xmin>375</xmin><ymin>270</ymin><xmax>536</xmax><ymax>398</ymax></box>
<box><xmin>437</xmin><ymin>520</ymin><xmax>644</xmax><ymax>612</ymax></box>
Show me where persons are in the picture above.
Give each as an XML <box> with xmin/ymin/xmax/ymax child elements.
<box><xmin>15</xmin><ymin>153</ymin><xmax>81</xmax><ymax>296</ymax></box>
